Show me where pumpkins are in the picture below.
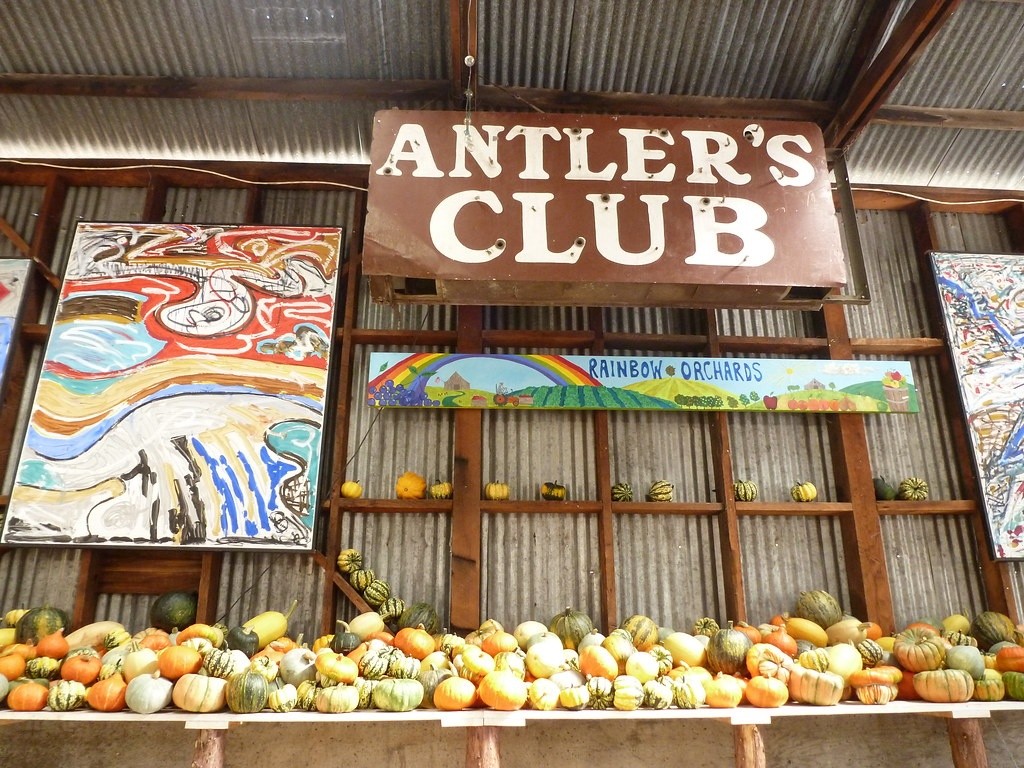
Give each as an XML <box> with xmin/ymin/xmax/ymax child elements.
<box><xmin>0</xmin><ymin>470</ymin><xmax>1024</xmax><ymax>712</ymax></box>
